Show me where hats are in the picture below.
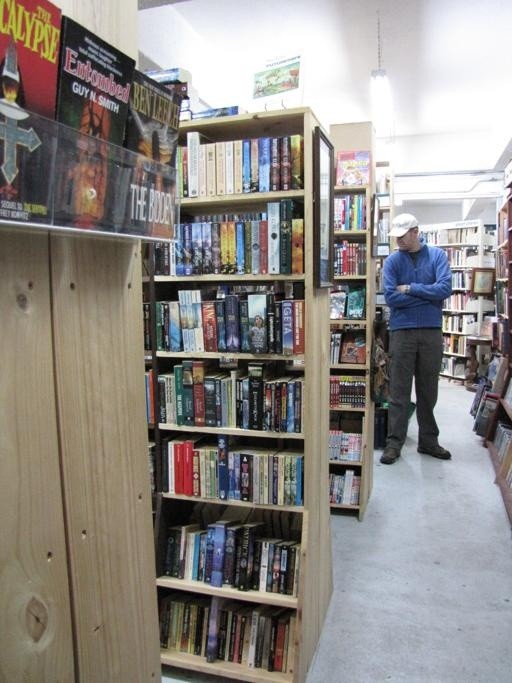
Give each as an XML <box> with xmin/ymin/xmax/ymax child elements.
<box><xmin>387</xmin><ymin>213</ymin><xmax>419</xmax><ymax>238</ymax></box>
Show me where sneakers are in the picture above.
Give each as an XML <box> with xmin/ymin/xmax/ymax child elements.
<box><xmin>416</xmin><ymin>443</ymin><xmax>452</xmax><ymax>459</ymax></box>
<box><xmin>381</xmin><ymin>447</ymin><xmax>401</xmax><ymax>465</ymax></box>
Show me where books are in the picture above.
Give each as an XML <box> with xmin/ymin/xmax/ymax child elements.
<box><xmin>420</xmin><ymin>199</ymin><xmax>512</xmax><ymax>528</ymax></box>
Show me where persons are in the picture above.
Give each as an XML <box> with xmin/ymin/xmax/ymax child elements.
<box><xmin>380</xmin><ymin>214</ymin><xmax>451</xmax><ymax>464</ymax></box>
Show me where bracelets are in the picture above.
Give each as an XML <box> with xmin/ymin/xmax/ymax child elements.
<box><xmin>404</xmin><ymin>284</ymin><xmax>409</xmax><ymax>294</ymax></box>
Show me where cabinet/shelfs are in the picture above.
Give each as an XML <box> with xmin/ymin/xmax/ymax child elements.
<box><xmin>330</xmin><ymin>184</ymin><xmax>391</xmax><ymax>524</ymax></box>
<box><xmin>414</xmin><ymin>193</ymin><xmax>512</xmax><ymax>524</ymax></box>
<box><xmin>143</xmin><ymin>107</ymin><xmax>335</xmax><ymax>683</ymax></box>
